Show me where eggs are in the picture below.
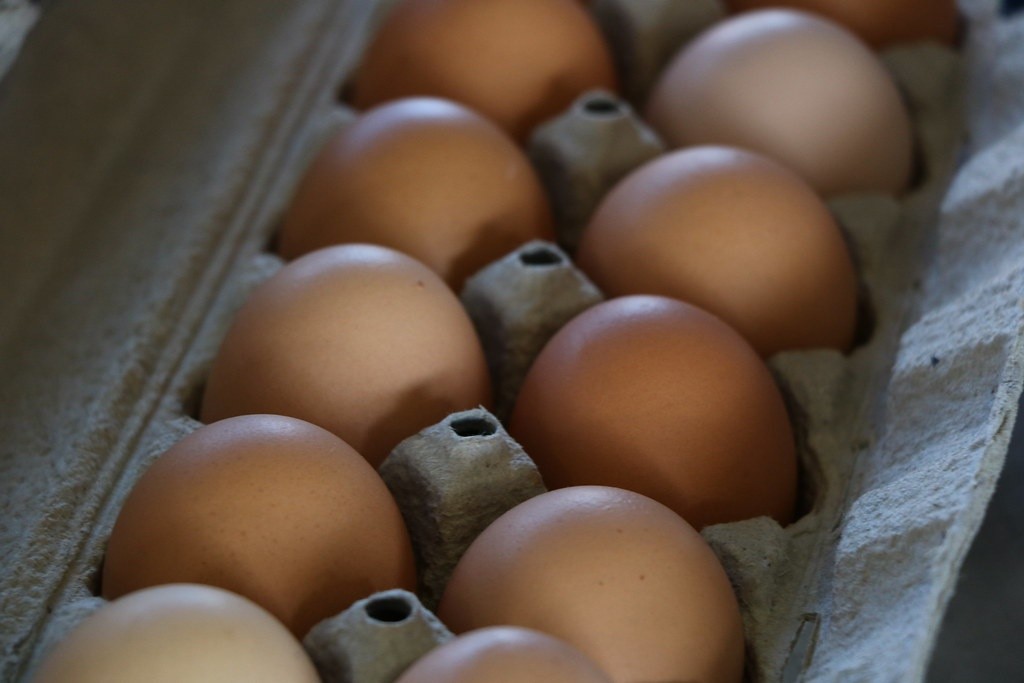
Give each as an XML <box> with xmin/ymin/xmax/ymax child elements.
<box><xmin>31</xmin><ymin>0</ymin><xmax>963</xmax><ymax>683</ymax></box>
